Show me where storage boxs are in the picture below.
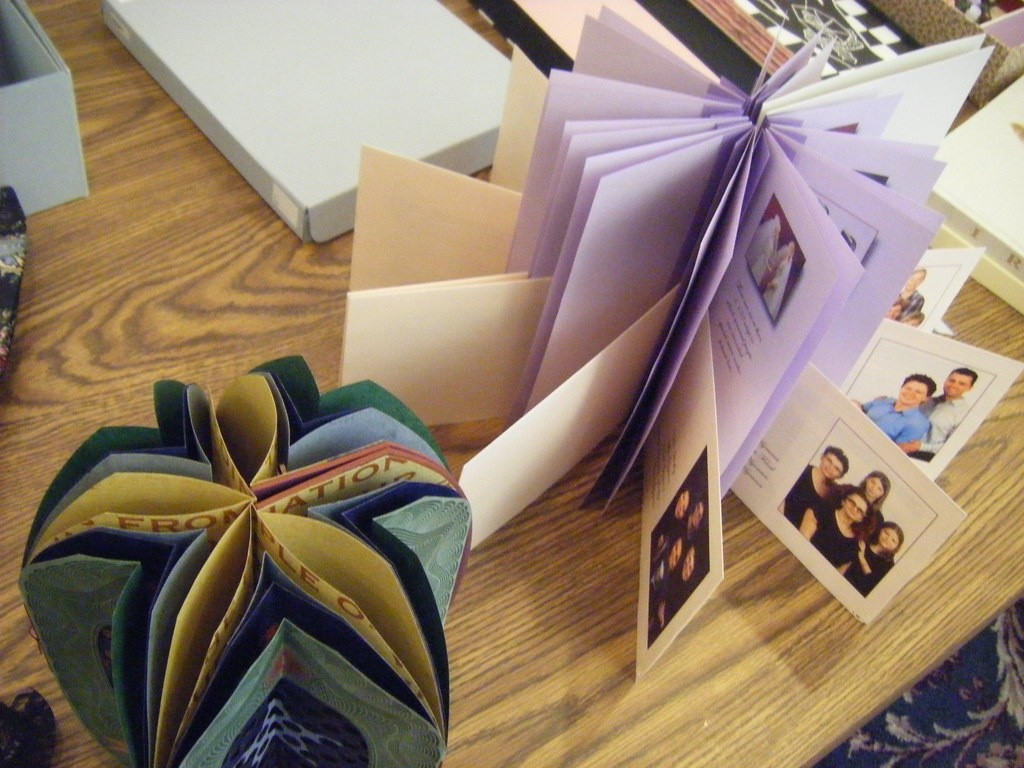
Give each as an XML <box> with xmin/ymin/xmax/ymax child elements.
<box><xmin>0</xmin><ymin>0</ymin><xmax>90</xmax><ymax>217</ymax></box>
<box><xmin>100</xmin><ymin>0</ymin><xmax>511</xmax><ymax>244</ymax></box>
<box><xmin>930</xmin><ymin>75</ymin><xmax>1024</xmax><ymax>317</ymax></box>
<box><xmin>873</xmin><ymin>0</ymin><xmax>1024</xmax><ymax>106</ymax></box>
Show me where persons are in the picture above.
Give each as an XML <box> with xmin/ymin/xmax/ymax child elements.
<box><xmin>778</xmin><ymin>368</ymin><xmax>978</xmax><ymax>598</ymax></box>
<box><xmin>745</xmin><ymin>214</ymin><xmax>796</xmax><ymax>325</ymax></box>
<box><xmin>649</xmin><ymin>488</ymin><xmax>706</xmax><ymax>647</ymax></box>
<box><xmin>885</xmin><ymin>268</ymin><xmax>927</xmax><ymax>328</ymax></box>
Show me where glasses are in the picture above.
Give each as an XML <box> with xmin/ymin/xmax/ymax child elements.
<box><xmin>848</xmin><ymin>497</ymin><xmax>867</xmax><ymax>518</ymax></box>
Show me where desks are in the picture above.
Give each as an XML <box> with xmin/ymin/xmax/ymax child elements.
<box><xmin>3</xmin><ymin>1</ymin><xmax>1024</xmax><ymax>767</ymax></box>
